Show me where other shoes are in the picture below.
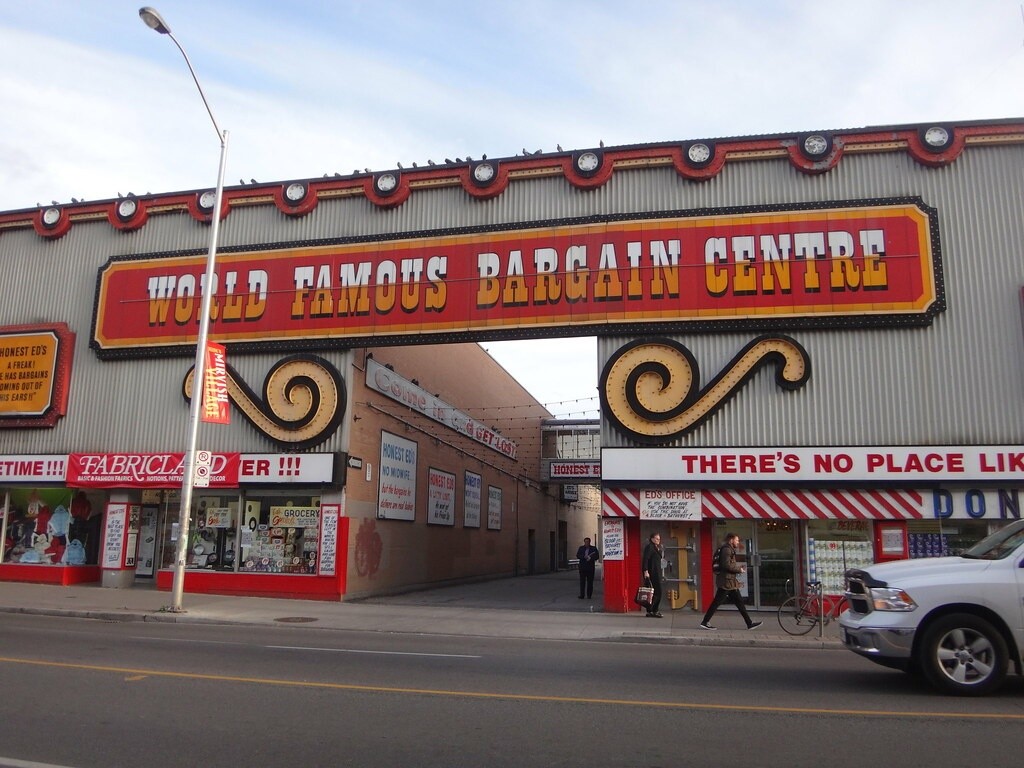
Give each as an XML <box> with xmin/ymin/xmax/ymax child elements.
<box><xmin>748</xmin><ymin>622</ymin><xmax>763</xmax><ymax>630</ymax></box>
<box><xmin>646</xmin><ymin>612</ymin><xmax>663</xmax><ymax>618</ymax></box>
<box><xmin>587</xmin><ymin>595</ymin><xmax>591</xmax><ymax>599</ymax></box>
<box><xmin>700</xmin><ymin>621</ymin><xmax>717</xmax><ymax>630</ymax></box>
<box><xmin>578</xmin><ymin>595</ymin><xmax>584</xmax><ymax>599</ymax></box>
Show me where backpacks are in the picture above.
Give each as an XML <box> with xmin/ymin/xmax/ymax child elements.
<box><xmin>713</xmin><ymin>546</ymin><xmax>734</xmax><ymax>574</ymax></box>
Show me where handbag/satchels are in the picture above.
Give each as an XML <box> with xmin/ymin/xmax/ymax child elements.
<box><xmin>635</xmin><ymin>577</ymin><xmax>654</xmax><ymax>606</ymax></box>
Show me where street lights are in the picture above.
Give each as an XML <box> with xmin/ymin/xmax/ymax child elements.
<box><xmin>140</xmin><ymin>5</ymin><xmax>225</xmax><ymax>613</ymax></box>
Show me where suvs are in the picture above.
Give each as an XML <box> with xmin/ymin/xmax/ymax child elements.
<box><xmin>837</xmin><ymin>518</ymin><xmax>1024</xmax><ymax>692</ymax></box>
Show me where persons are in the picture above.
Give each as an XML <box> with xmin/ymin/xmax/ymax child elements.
<box><xmin>642</xmin><ymin>532</ymin><xmax>664</xmax><ymax>617</ymax></box>
<box><xmin>699</xmin><ymin>533</ymin><xmax>763</xmax><ymax>630</ymax></box>
<box><xmin>576</xmin><ymin>538</ymin><xmax>599</xmax><ymax>599</ymax></box>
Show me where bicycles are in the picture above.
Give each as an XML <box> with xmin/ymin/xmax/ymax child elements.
<box><xmin>777</xmin><ymin>580</ymin><xmax>849</xmax><ymax>636</ymax></box>
